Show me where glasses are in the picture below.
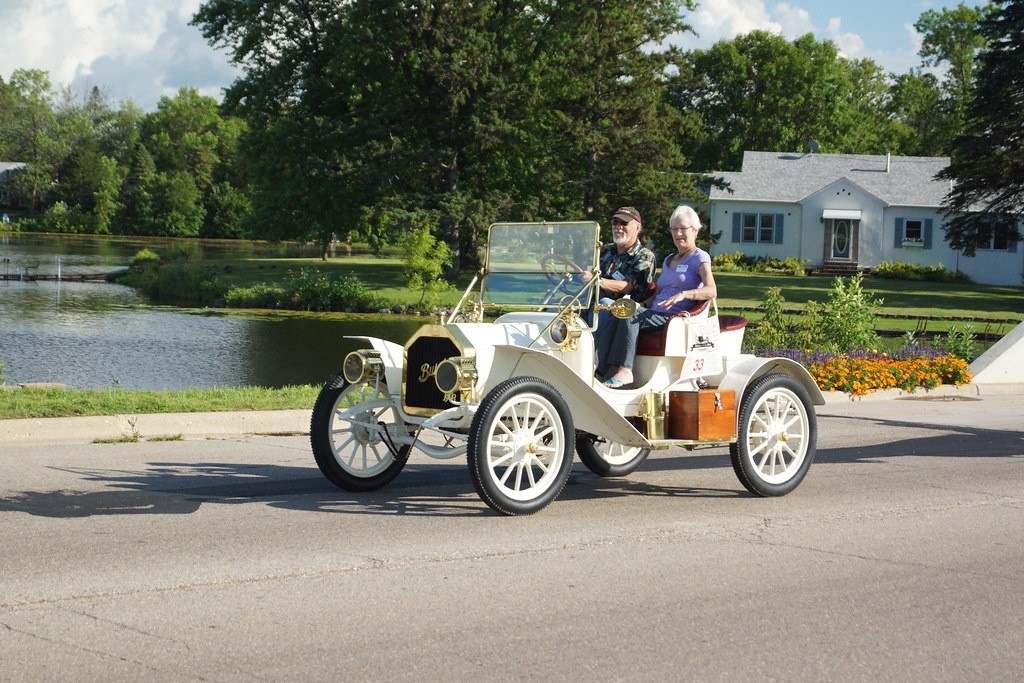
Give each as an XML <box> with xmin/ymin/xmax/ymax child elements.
<box><xmin>672</xmin><ymin>226</ymin><xmax>692</xmax><ymax>231</ymax></box>
<box><xmin>611</xmin><ymin>218</ymin><xmax>634</xmax><ymax>226</ymax></box>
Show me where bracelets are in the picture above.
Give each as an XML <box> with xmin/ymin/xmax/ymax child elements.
<box><xmin>666</xmin><ymin>299</ymin><xmax>669</xmax><ymax>301</ymax></box>
<box><xmin>594</xmin><ymin>277</ymin><xmax>602</xmax><ymax>289</ymax></box>
<box><xmin>693</xmin><ymin>290</ymin><xmax>698</xmax><ymax>300</ymax></box>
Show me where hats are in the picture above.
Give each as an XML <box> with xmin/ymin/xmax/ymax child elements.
<box><xmin>612</xmin><ymin>207</ymin><xmax>641</xmax><ymax>223</ymax></box>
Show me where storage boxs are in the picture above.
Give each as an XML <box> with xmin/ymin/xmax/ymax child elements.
<box><xmin>668</xmin><ymin>390</ymin><xmax>737</xmax><ymax>441</ymax></box>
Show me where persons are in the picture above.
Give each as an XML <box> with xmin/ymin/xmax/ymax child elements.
<box><xmin>596</xmin><ymin>205</ymin><xmax>716</xmax><ymax>389</ymax></box>
<box><xmin>548</xmin><ymin>207</ymin><xmax>656</xmax><ymax>348</ymax></box>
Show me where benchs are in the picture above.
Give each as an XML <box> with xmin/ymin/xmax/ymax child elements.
<box><xmin>635</xmin><ymin>282</ymin><xmax>711</xmax><ymax>357</ymax></box>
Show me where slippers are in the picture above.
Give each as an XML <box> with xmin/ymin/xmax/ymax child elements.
<box><xmin>602</xmin><ymin>377</ymin><xmax>637</xmax><ymax>389</ymax></box>
